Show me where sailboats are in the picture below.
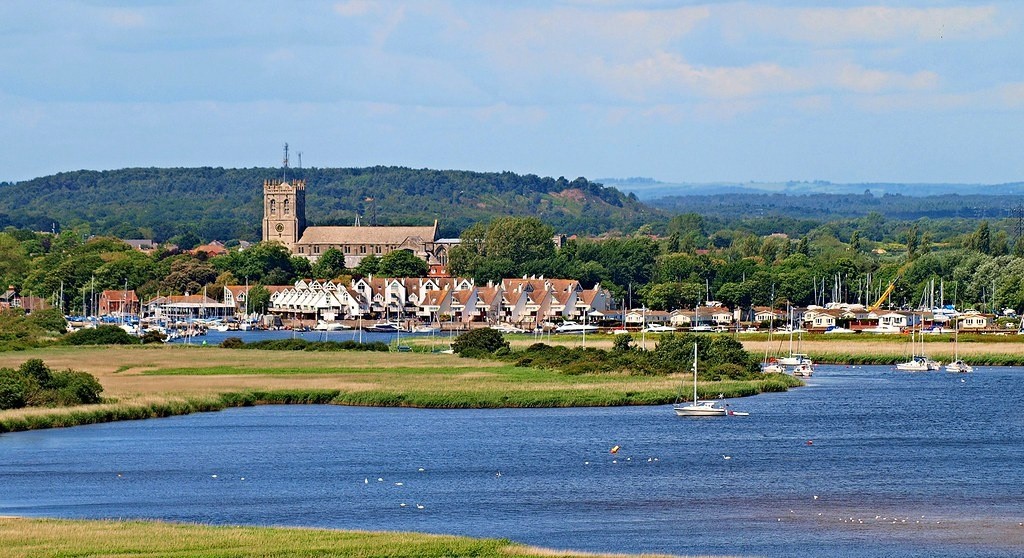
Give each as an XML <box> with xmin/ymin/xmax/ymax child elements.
<box><xmin>944</xmin><ymin>316</ymin><xmax>974</xmax><ymax>372</ymax></box>
<box><xmin>440</xmin><ymin>308</ymin><xmax>456</xmax><ymax>354</ymax></box>
<box><xmin>740</xmin><ymin>308</ymin><xmax>765</xmax><ymax>334</ymax></box>
<box><xmin>61</xmin><ymin>277</ymin><xmax>443</xmax><ymax>343</ymax></box>
<box><xmin>759</xmin><ymin>319</ymin><xmax>785</xmax><ymax>374</ymax></box>
<box><xmin>690</xmin><ymin>304</ymin><xmax>713</xmax><ymax>333</ymax></box>
<box><xmin>773</xmin><ymin>271</ymin><xmax>1024</xmax><ymax>336</ymax></box>
<box><xmin>673</xmin><ymin>342</ymin><xmax>726</xmax><ymax>417</ymax></box>
<box><xmin>897</xmin><ymin>312</ymin><xmax>942</xmax><ymax>371</ymax></box>
<box><xmin>793</xmin><ymin>313</ymin><xmax>815</xmax><ymax>378</ymax></box>
<box><xmin>609</xmin><ymin>284</ymin><xmax>642</xmax><ymax>334</ymax></box>
<box><xmin>775</xmin><ymin>306</ymin><xmax>812</xmax><ymax>366</ymax></box>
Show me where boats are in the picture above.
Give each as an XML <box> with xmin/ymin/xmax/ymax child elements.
<box><xmin>641</xmin><ymin>322</ymin><xmax>677</xmax><ymax>333</ymax></box>
<box><xmin>557</xmin><ymin>321</ymin><xmax>600</xmax><ymax>334</ymax></box>
<box><xmin>714</xmin><ymin>325</ymin><xmax>728</xmax><ymax>333</ymax></box>
<box><xmin>535</xmin><ymin>315</ymin><xmax>564</xmax><ymax>334</ymax></box>
<box><xmin>488</xmin><ymin>302</ymin><xmax>529</xmax><ymax>334</ymax></box>
<box><xmin>731</xmin><ymin>412</ymin><xmax>749</xmax><ymax>417</ymax></box>
